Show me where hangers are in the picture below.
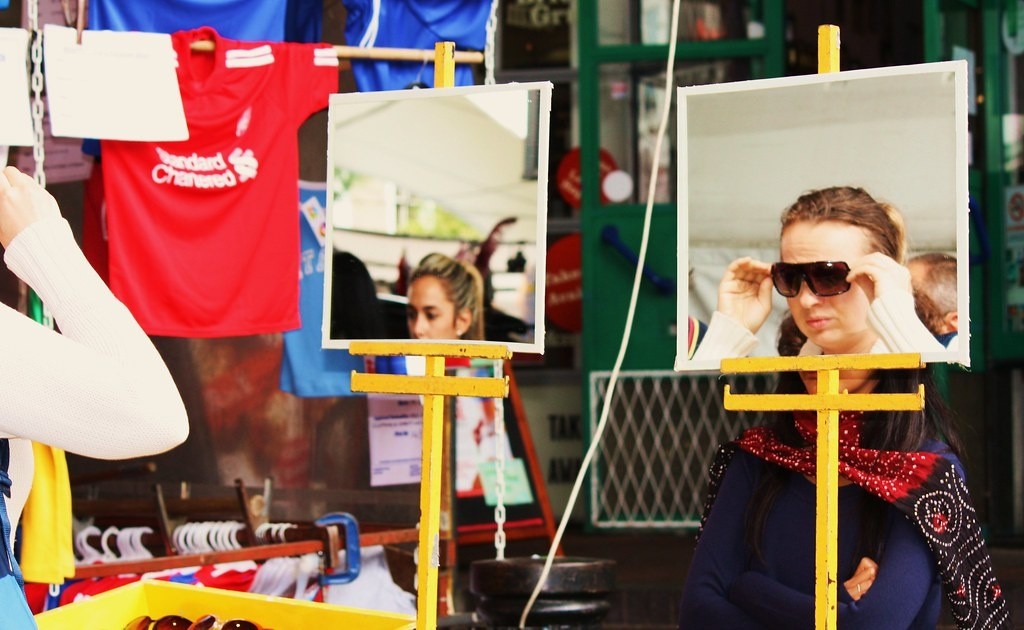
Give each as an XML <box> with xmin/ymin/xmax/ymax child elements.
<box><xmin>70</xmin><ymin>516</ymin><xmax>327</xmax><ymax>603</ymax></box>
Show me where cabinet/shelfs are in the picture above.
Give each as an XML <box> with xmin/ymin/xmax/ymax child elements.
<box><xmin>489</xmin><ymin>0</ymin><xmax>787</xmax><ymax>533</ymax></box>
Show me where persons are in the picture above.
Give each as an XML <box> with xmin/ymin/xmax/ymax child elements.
<box><xmin>688</xmin><ymin>185</ymin><xmax>958</xmax><ymax>362</ymax></box>
<box><xmin>0</xmin><ymin>165</ymin><xmax>190</xmax><ymax>630</ymax></box>
<box><xmin>678</xmin><ymin>362</ymin><xmax>970</xmax><ymax>630</ymax></box>
<box><xmin>406</xmin><ymin>252</ymin><xmax>486</xmax><ymax>340</ymax></box>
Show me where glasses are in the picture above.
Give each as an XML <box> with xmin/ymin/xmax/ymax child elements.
<box><xmin>771</xmin><ymin>261</ymin><xmax>852</xmax><ymax>297</ymax></box>
<box><xmin>188</xmin><ymin>614</ymin><xmax>270</xmax><ymax>630</ymax></box>
<box><xmin>124</xmin><ymin>616</ymin><xmax>192</xmax><ymax>630</ymax></box>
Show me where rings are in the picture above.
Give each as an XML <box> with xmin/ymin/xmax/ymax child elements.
<box><xmin>857</xmin><ymin>584</ymin><xmax>860</xmax><ymax>593</ymax></box>
